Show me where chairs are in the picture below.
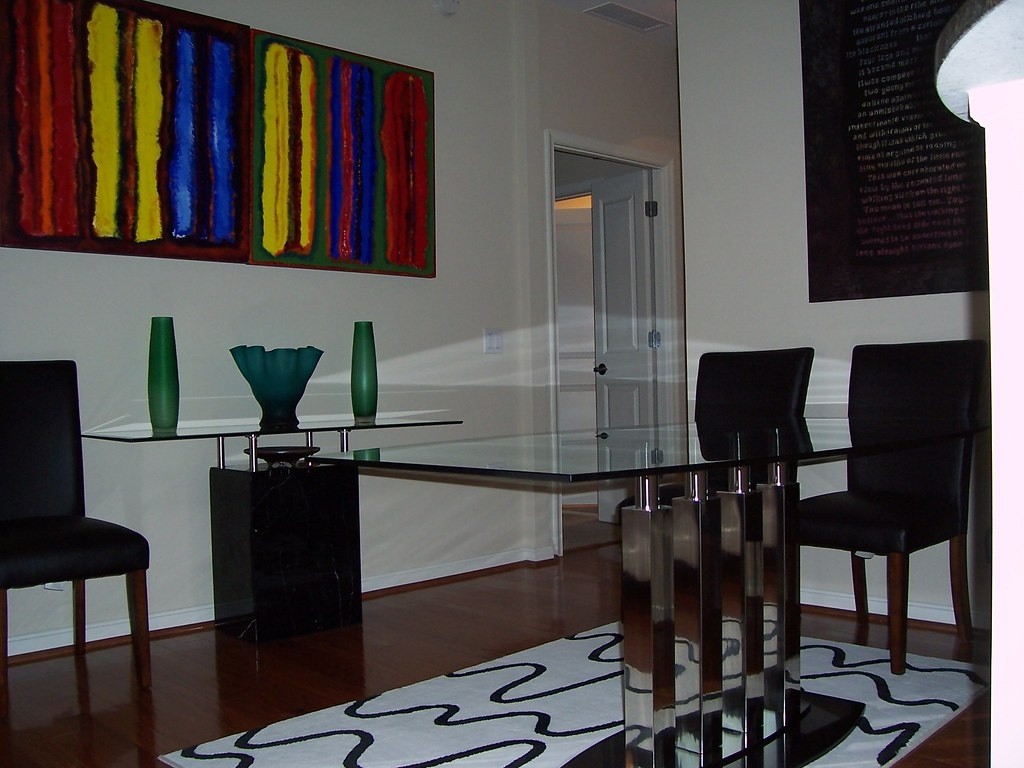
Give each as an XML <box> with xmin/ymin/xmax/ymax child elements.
<box><xmin>799</xmin><ymin>338</ymin><xmax>989</xmax><ymax>675</ymax></box>
<box><xmin>0</xmin><ymin>359</ymin><xmax>151</xmax><ymax>723</ymax></box>
<box><xmin>617</xmin><ymin>343</ymin><xmax>815</xmax><ymax>542</ymax></box>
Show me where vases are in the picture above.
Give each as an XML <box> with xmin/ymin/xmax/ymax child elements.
<box><xmin>229</xmin><ymin>343</ymin><xmax>325</xmax><ymax>429</ymax></box>
<box><xmin>350</xmin><ymin>320</ymin><xmax>378</xmax><ymax>423</ymax></box>
<box><xmin>147</xmin><ymin>316</ymin><xmax>180</xmax><ymax>435</ymax></box>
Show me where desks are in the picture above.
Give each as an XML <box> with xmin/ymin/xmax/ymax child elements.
<box><xmin>305</xmin><ymin>417</ymin><xmax>854</xmax><ymax>768</ymax></box>
<box><xmin>81</xmin><ymin>418</ymin><xmax>465</xmax><ymax>645</ymax></box>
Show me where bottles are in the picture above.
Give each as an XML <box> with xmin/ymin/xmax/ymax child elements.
<box><xmin>352</xmin><ymin>321</ymin><xmax>377</xmax><ymax>423</ymax></box>
<box><xmin>149</xmin><ymin>316</ymin><xmax>179</xmax><ymax>433</ymax></box>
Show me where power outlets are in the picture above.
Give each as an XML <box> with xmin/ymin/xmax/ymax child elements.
<box><xmin>44</xmin><ymin>581</ymin><xmax>66</xmax><ymax>591</ymax></box>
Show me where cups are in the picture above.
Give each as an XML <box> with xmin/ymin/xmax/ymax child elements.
<box><xmin>231</xmin><ymin>345</ymin><xmax>324</xmax><ymax>426</ymax></box>
<box><xmin>352</xmin><ymin>448</ymin><xmax>381</xmax><ymax>461</ymax></box>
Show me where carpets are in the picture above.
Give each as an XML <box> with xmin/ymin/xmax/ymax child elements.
<box><xmin>157</xmin><ymin>614</ymin><xmax>991</xmax><ymax>768</ymax></box>
